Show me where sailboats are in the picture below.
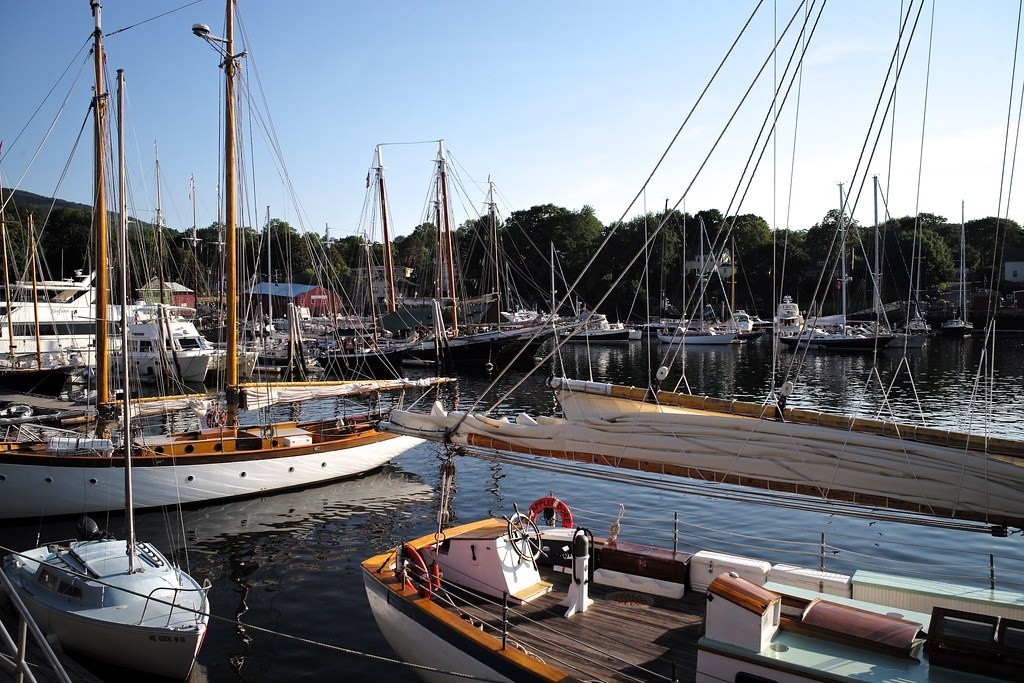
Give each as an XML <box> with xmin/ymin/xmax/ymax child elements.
<box><xmin>0</xmin><ymin>0</ymin><xmax>1024</xmax><ymax>683</ymax></box>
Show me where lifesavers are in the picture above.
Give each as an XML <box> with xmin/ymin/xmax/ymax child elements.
<box><xmin>264</xmin><ymin>425</ymin><xmax>274</xmax><ymax>439</ymax></box>
<box><xmin>207</xmin><ymin>409</ymin><xmax>227</xmax><ymax>429</ymax></box>
<box><xmin>527</xmin><ymin>496</ymin><xmax>572</xmax><ymax>528</ymax></box>
<box><xmin>396</xmin><ymin>544</ymin><xmax>432</xmax><ymax>599</ymax></box>
